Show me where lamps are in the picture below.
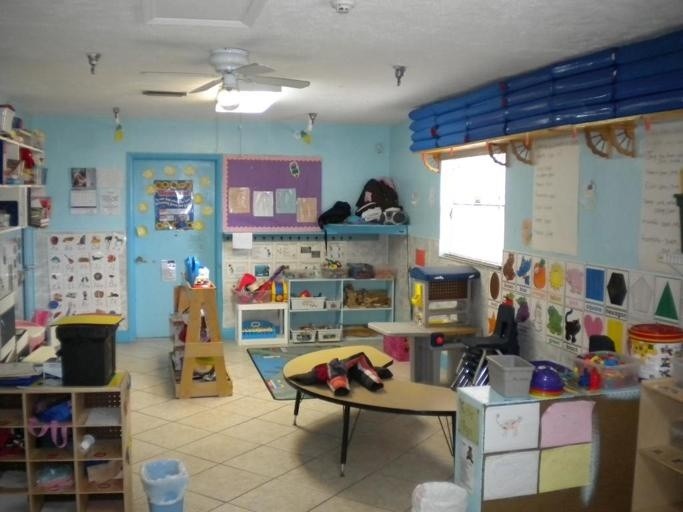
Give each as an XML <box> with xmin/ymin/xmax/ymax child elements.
<box><xmin>217</xmin><ymin>76</ymin><xmax>242</xmax><ymax>111</ymax></box>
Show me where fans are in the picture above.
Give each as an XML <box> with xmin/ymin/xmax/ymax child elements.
<box><xmin>137</xmin><ymin>46</ymin><xmax>311</xmax><ymax>95</ymax></box>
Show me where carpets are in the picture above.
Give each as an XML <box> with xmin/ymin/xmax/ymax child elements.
<box><xmin>245</xmin><ymin>345</ymin><xmax>339</xmax><ymax>400</ymax></box>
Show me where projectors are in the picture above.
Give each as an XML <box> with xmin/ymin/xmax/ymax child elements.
<box><xmin>0</xmin><ymin>104</ymin><xmax>22</xmax><ymax>135</ymax></box>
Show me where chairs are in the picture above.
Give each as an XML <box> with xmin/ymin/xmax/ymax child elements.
<box><xmin>449</xmin><ymin>301</ymin><xmax>522</xmax><ymax>390</ymax></box>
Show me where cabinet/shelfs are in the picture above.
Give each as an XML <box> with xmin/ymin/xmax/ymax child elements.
<box><xmin>0</xmin><ymin>136</ymin><xmax>48</xmax><ymax>234</ymax></box>
<box><xmin>0</xmin><ymin>371</ymin><xmax>134</xmax><ymax>512</ymax></box>
<box><xmin>406</xmin><ymin>263</ymin><xmax>480</xmax><ymax>328</ymax></box>
<box><xmin>453</xmin><ymin>385</ymin><xmax>640</xmax><ymax>512</ymax></box>
<box><xmin>167</xmin><ymin>281</ymin><xmax>234</xmax><ymax>400</ymax></box>
<box><xmin>631</xmin><ymin>378</ymin><xmax>682</xmax><ymax>511</ymax></box>
<box><xmin>235</xmin><ymin>273</ymin><xmax>397</xmax><ymax>348</ymax></box>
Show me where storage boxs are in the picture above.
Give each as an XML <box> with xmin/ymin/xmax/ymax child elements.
<box><xmin>317</xmin><ymin>327</ymin><xmax>342</xmax><ymax>342</ymax></box>
<box><xmin>54</xmin><ymin>324</ymin><xmax>117</xmax><ymax>387</ymax></box>
<box><xmin>484</xmin><ymin>351</ymin><xmax>537</xmax><ymax>399</ymax></box>
<box><xmin>289</xmin><ymin>327</ymin><xmax>316</xmax><ymax>343</ymax></box>
<box><xmin>570</xmin><ymin>349</ymin><xmax>642</xmax><ymax>391</ymax></box>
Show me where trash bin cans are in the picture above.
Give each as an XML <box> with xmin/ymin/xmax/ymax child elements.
<box><xmin>141</xmin><ymin>458</ymin><xmax>189</xmax><ymax>512</ymax></box>
<box><xmin>411</xmin><ymin>481</ymin><xmax>468</xmax><ymax>512</ymax></box>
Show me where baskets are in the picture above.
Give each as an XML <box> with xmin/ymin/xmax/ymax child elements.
<box><xmin>429</xmin><ymin>280</ymin><xmax>465</xmax><ymax>299</ymax></box>
<box><xmin>313</xmin><ymin>323</ymin><xmax>343</xmax><ymax>342</ymax></box>
<box><xmin>326</xmin><ymin>300</ymin><xmax>341</xmax><ymax>310</ymax></box>
<box><xmin>291</xmin><ymin>324</ymin><xmax>317</xmax><ymax>343</ymax></box>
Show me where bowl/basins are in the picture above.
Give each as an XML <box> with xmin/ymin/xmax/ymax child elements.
<box><xmin>530</xmin><ymin>368</ymin><xmax>565</xmax><ymax>398</ymax></box>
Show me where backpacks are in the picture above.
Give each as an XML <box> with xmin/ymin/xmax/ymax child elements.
<box><xmin>27</xmin><ymin>396</ymin><xmax>73</xmax><ymax>448</ymax></box>
<box><xmin>36</xmin><ymin>464</ymin><xmax>74</xmax><ymax>493</ymax></box>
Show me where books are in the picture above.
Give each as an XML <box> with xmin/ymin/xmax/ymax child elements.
<box><xmin>31</xmin><ymin>221</ymin><xmax>50</xmax><ymax>228</ymax></box>
<box><xmin>31</xmin><ymin>215</ymin><xmax>50</xmax><ymax>223</ymax></box>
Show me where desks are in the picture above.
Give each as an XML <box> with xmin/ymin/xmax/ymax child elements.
<box><xmin>365</xmin><ymin>321</ymin><xmax>479</xmax><ymax>383</ymax></box>
<box><xmin>281</xmin><ymin>340</ymin><xmax>462</xmax><ymax>477</ymax></box>
